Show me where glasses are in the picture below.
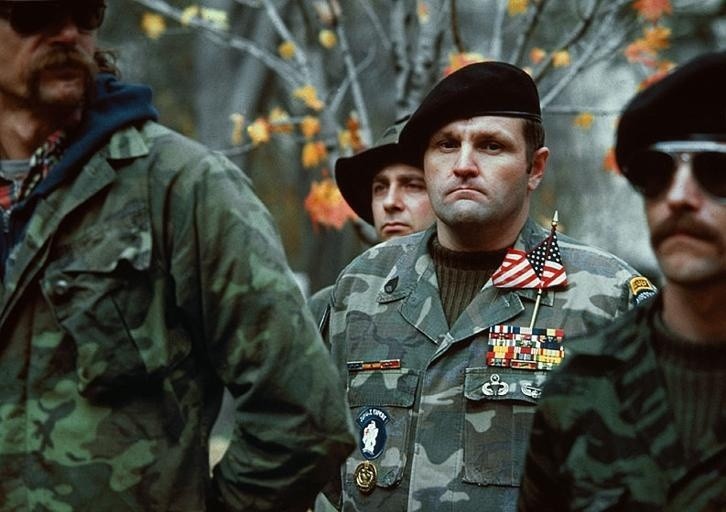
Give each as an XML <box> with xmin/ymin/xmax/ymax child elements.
<box><xmin>0</xmin><ymin>1</ymin><xmax>107</xmax><ymax>34</ymax></box>
<box><xmin>627</xmin><ymin>150</ymin><xmax>726</xmax><ymax>198</ymax></box>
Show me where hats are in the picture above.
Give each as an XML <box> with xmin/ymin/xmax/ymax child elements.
<box><xmin>334</xmin><ymin>61</ymin><xmax>543</xmax><ymax>228</ymax></box>
<box><xmin>615</xmin><ymin>51</ymin><xmax>725</xmax><ymax>177</ymax></box>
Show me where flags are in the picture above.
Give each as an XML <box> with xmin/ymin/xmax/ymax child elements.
<box><xmin>492</xmin><ymin>235</ymin><xmax>567</xmax><ymax>289</ymax></box>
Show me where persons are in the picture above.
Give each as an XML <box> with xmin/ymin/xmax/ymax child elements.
<box><xmin>308</xmin><ymin>112</ymin><xmax>437</xmax><ymax>512</ymax></box>
<box><xmin>0</xmin><ymin>0</ymin><xmax>356</xmax><ymax>511</ymax></box>
<box><xmin>322</xmin><ymin>61</ymin><xmax>661</xmax><ymax>512</ymax></box>
<box><xmin>517</xmin><ymin>49</ymin><xmax>726</xmax><ymax>512</ymax></box>
